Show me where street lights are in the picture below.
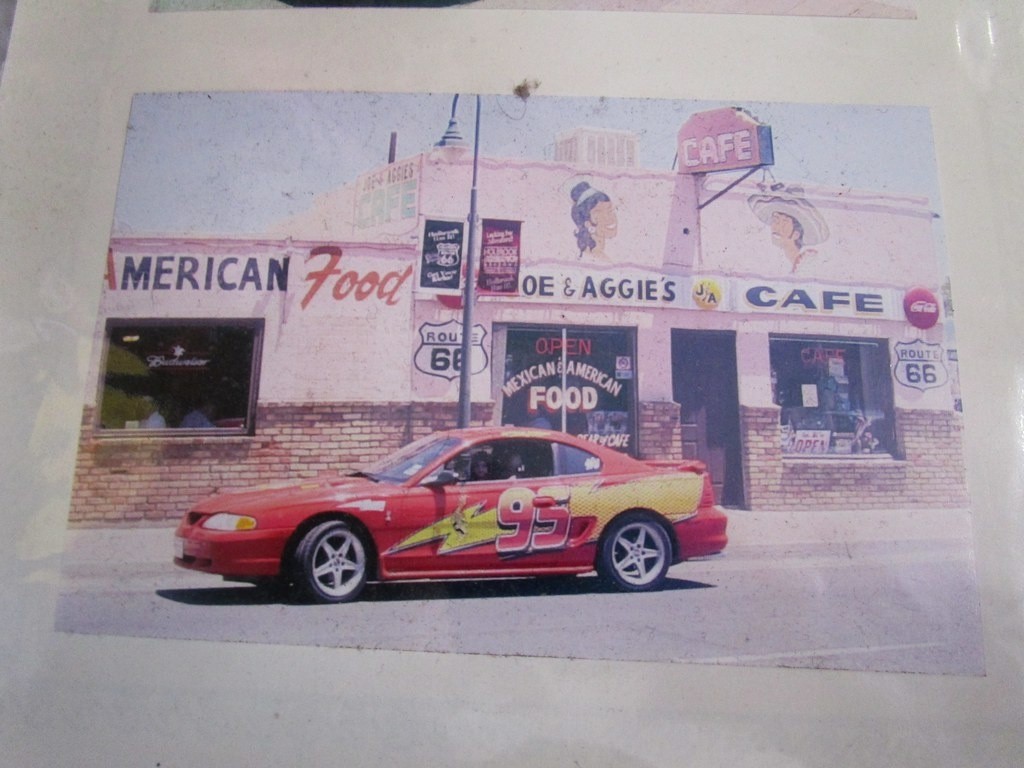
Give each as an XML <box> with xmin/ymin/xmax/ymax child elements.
<box><xmin>437</xmin><ymin>93</ymin><xmax>479</xmax><ymax>426</ymax></box>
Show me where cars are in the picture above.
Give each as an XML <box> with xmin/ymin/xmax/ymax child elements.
<box><xmin>172</xmin><ymin>426</ymin><xmax>728</xmax><ymax>604</ymax></box>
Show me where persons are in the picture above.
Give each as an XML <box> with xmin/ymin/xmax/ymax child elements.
<box><xmin>178</xmin><ymin>403</ymin><xmax>219</xmax><ymax>429</ymax></box>
<box><xmin>144</xmin><ymin>400</ymin><xmax>175</xmax><ymax>429</ymax></box>
<box><xmin>471</xmin><ymin>451</ymin><xmax>495</xmax><ymax>480</ymax></box>
<box><xmin>501</xmin><ymin>451</ymin><xmax>527</xmax><ymax>479</ymax></box>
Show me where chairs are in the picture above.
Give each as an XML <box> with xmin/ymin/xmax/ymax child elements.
<box><xmin>497</xmin><ymin>451</ymin><xmax>525</xmax><ymax>478</ymax></box>
